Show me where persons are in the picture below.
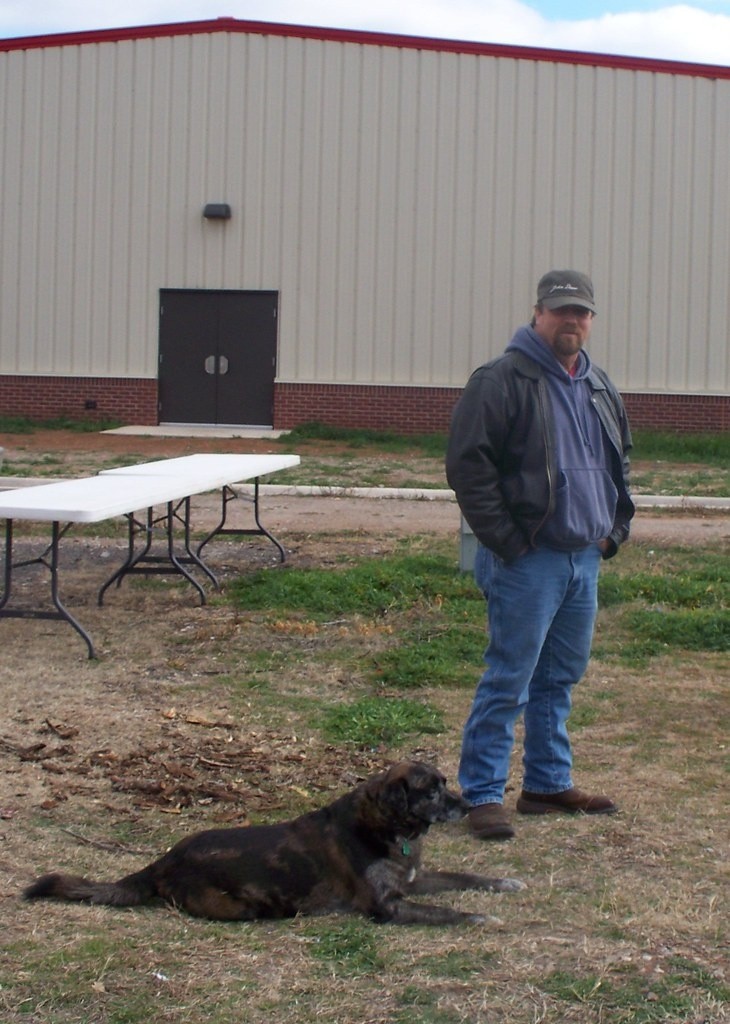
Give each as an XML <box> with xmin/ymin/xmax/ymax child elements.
<box><xmin>446</xmin><ymin>269</ymin><xmax>638</xmax><ymax>839</ymax></box>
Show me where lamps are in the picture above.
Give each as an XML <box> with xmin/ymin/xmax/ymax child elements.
<box><xmin>204</xmin><ymin>203</ymin><xmax>230</xmax><ymax>218</ymax></box>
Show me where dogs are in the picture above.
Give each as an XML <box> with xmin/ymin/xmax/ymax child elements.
<box><xmin>22</xmin><ymin>757</ymin><xmax>529</xmax><ymax>924</ymax></box>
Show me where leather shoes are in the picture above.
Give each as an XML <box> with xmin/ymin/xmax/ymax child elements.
<box><xmin>516</xmin><ymin>787</ymin><xmax>617</xmax><ymax>814</ymax></box>
<box><xmin>468</xmin><ymin>802</ymin><xmax>514</xmax><ymax>839</ymax></box>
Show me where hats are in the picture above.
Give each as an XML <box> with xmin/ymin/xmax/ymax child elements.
<box><xmin>537</xmin><ymin>268</ymin><xmax>598</xmax><ymax>314</ymax></box>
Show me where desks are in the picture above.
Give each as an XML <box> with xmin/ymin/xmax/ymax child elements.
<box><xmin>0</xmin><ymin>454</ymin><xmax>301</xmax><ymax>660</ymax></box>
